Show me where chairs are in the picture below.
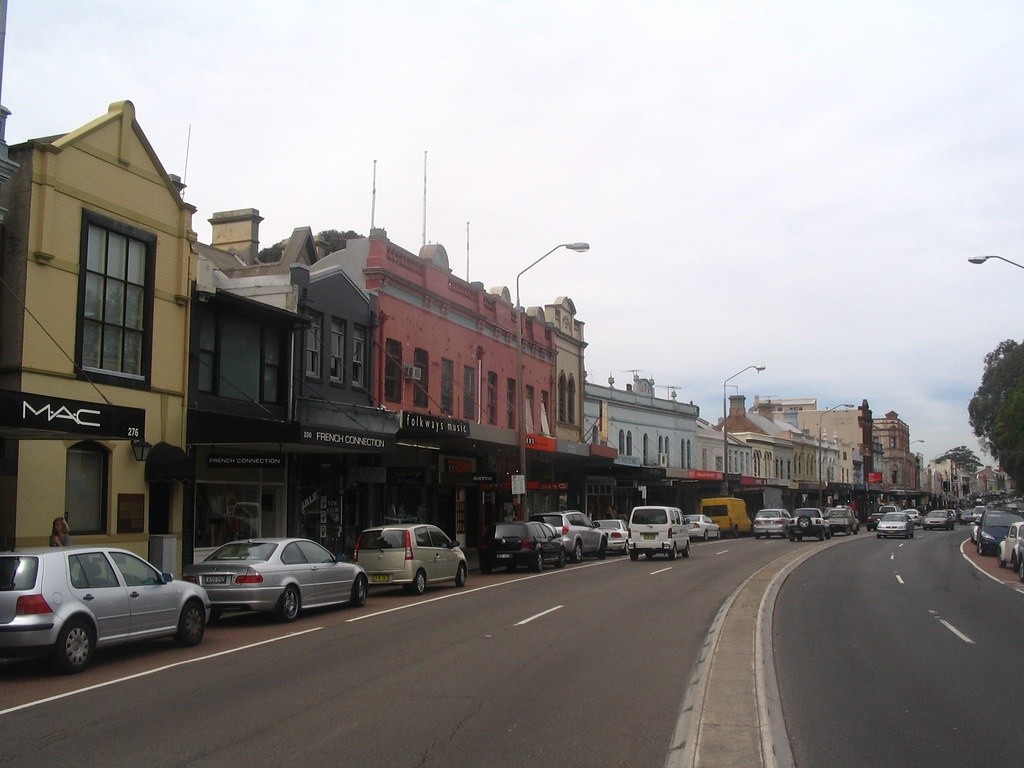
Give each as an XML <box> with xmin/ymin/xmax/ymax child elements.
<box><xmin>76</xmin><ymin>563</ymin><xmax>106</xmax><ymax>587</ymax></box>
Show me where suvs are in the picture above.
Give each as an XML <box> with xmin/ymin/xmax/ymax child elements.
<box><xmin>528</xmin><ymin>509</ymin><xmax>608</xmax><ymax>565</ymax></box>
<box><xmin>787</xmin><ymin>508</ymin><xmax>832</xmax><ymax>543</ymax></box>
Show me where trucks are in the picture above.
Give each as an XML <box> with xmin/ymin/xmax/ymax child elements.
<box><xmin>700</xmin><ymin>498</ymin><xmax>753</xmax><ymax>539</ymax></box>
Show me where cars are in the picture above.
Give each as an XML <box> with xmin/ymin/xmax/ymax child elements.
<box><xmin>683</xmin><ymin>514</ymin><xmax>721</xmax><ymax>542</ymax></box>
<box><xmin>975</xmin><ymin>509</ymin><xmax>1024</xmax><ymax>557</ymax></box>
<box><xmin>997</xmin><ymin>521</ymin><xmax>1024</xmax><ymax>583</ymax></box>
<box><xmin>0</xmin><ymin>546</ymin><xmax>212</xmax><ymax>675</ymax></box>
<box><xmin>752</xmin><ymin>509</ymin><xmax>793</xmax><ymax>539</ymax></box>
<box><xmin>476</xmin><ymin>521</ymin><xmax>567</xmax><ymax>576</ymax></box>
<box><xmin>182</xmin><ymin>539</ymin><xmax>370</xmax><ymax>623</ymax></box>
<box><xmin>353</xmin><ymin>524</ymin><xmax>468</xmax><ymax>596</ymax></box>
<box><xmin>825</xmin><ymin>505</ymin><xmax>924</xmax><ymax>539</ymax></box>
<box><xmin>922</xmin><ymin>505</ymin><xmax>987</xmax><ymax>545</ymax></box>
<box><xmin>593</xmin><ymin>520</ymin><xmax>629</xmax><ymax>556</ymax></box>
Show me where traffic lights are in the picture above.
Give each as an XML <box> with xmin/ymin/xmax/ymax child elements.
<box><xmin>943</xmin><ymin>481</ymin><xmax>948</xmax><ymax>492</ymax></box>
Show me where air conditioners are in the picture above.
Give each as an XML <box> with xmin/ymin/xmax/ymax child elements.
<box><xmin>405</xmin><ymin>366</ymin><xmax>422</xmax><ymax>379</ymax></box>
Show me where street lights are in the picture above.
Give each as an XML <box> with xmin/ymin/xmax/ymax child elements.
<box><xmin>517</xmin><ymin>243</ymin><xmax>590</xmax><ymax>522</ymax></box>
<box><xmin>819</xmin><ymin>403</ymin><xmax>854</xmax><ymax>509</ymax></box>
<box><xmin>723</xmin><ymin>365</ymin><xmax>766</xmax><ymax>496</ymax></box>
<box><xmin>904</xmin><ymin>440</ymin><xmax>925</xmax><ymax>510</ymax></box>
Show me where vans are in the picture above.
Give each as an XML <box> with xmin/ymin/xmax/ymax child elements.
<box><xmin>628</xmin><ymin>506</ymin><xmax>690</xmax><ymax>560</ymax></box>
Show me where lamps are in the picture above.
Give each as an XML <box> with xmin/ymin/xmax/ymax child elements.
<box><xmin>131</xmin><ymin>437</ymin><xmax>152</xmax><ymax>461</ymax></box>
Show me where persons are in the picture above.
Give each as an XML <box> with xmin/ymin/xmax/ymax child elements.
<box><xmin>49</xmin><ymin>517</ymin><xmax>71</xmax><ymax>547</ymax></box>
<box><xmin>587</xmin><ymin>505</ymin><xmax>594</xmax><ymax>521</ymax></box>
<box><xmin>605</xmin><ymin>505</ymin><xmax>616</xmax><ymax>519</ymax></box>
